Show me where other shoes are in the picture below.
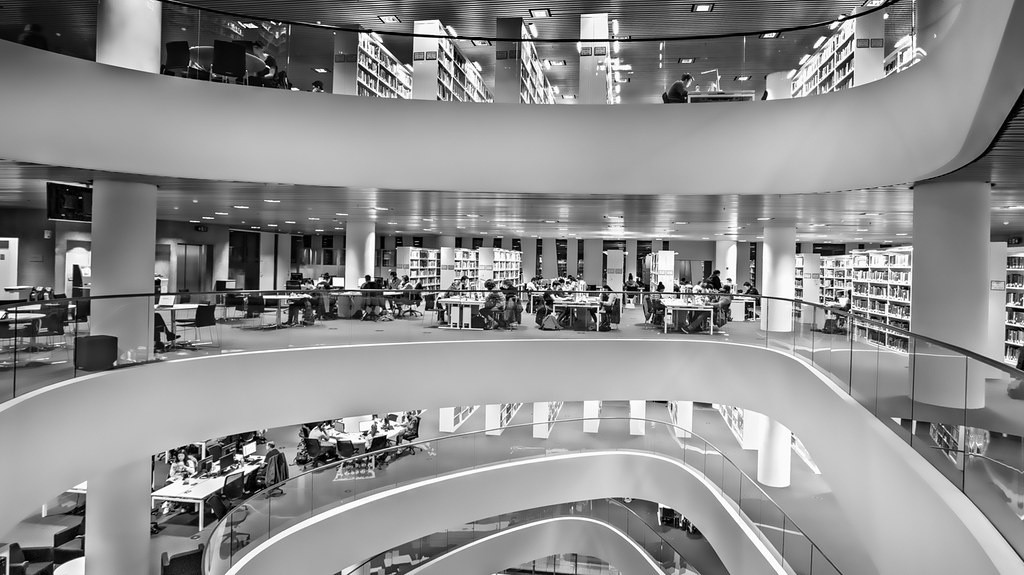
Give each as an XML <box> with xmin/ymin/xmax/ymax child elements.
<box><xmin>155</xmin><ymin>342</ymin><xmax>166</xmax><ymax>348</ymax></box>
<box><xmin>165</xmin><ymin>332</ymin><xmax>181</xmax><ymax>341</ymax></box>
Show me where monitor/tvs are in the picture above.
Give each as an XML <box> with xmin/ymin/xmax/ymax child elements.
<box><xmin>44</xmin><ymin>181</ymin><xmax>93</xmax><ymax>223</ymax></box>
<box><xmin>334</xmin><ymin>421</ymin><xmax>345</xmax><ymax>433</ymax></box>
<box><xmin>359</xmin><ymin>421</ymin><xmax>374</xmax><ymax>431</ymax></box>
<box><xmin>222</xmin><ymin>442</ymin><xmax>236</xmax><ymax>457</ymax></box>
<box><xmin>197</xmin><ymin>454</ymin><xmax>213</xmax><ymax>472</ymax></box>
<box><xmin>357</xmin><ymin>277</ymin><xmax>374</xmax><ymax>288</ymax></box>
<box><xmin>225</xmin><ymin>281</ymin><xmax>236</xmax><ymax>289</ymax></box>
<box><xmin>331</xmin><ymin>276</ymin><xmax>346</xmax><ymax>288</ymax></box>
<box><xmin>219</xmin><ymin>453</ymin><xmax>234</xmax><ymax>471</ymax></box>
<box><xmin>242</xmin><ymin>440</ymin><xmax>257</xmax><ymax>457</ymax></box>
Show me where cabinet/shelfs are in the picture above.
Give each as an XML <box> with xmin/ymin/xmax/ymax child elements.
<box><xmin>489</xmin><ymin>14</ymin><xmax>558</xmax><ymax>104</ymax></box>
<box><xmin>438</xmin><ymin>407</ymin><xmax>823</xmax><ymax>478</ymax></box>
<box><xmin>391</xmin><ymin>241</ymin><xmax>527</xmax><ymax>297</ymax></box>
<box><xmin>415</xmin><ymin>16</ymin><xmax>494</xmax><ymax>102</ymax></box>
<box><xmin>576</xmin><ymin>14</ymin><xmax>626</xmax><ymax>105</ymax></box>
<box><xmin>791</xmin><ymin>5</ymin><xmax>885</xmax><ymax>97</ymax></box>
<box><xmin>332</xmin><ymin>21</ymin><xmax>414</xmax><ymax>99</ymax></box>
<box><xmin>647</xmin><ymin>248</ymin><xmax>677</xmax><ymax>300</ymax></box>
<box><xmin>795</xmin><ymin>236</ymin><xmax>1023</xmax><ymax>377</ymax></box>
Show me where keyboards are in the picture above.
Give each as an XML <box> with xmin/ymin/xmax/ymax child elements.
<box><xmin>248</xmin><ymin>458</ymin><xmax>262</xmax><ymax>465</ymax></box>
<box><xmin>191</xmin><ymin>471</ymin><xmax>202</xmax><ymax>479</ymax></box>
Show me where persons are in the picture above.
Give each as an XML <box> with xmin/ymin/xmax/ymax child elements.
<box><xmin>526</xmin><ymin>274</ymin><xmax>587</xmax><ymax>329</ymax></box>
<box><xmin>263</xmin><ymin>442</ymin><xmax>279</xmax><ymax>463</ymax></box>
<box><xmin>316</xmin><ymin>272</ymin><xmax>337</xmax><ymax>320</ymax></box>
<box><xmin>281</xmin><ymin>277</ymin><xmax>315</xmax><ymax>327</ymax></box>
<box><xmin>306</xmin><ymin>411</ymin><xmax>419</xmax><ymax>462</ymax></box>
<box><xmin>674</xmin><ymin>277</ymin><xmax>703</xmax><ymax>299</ymax></box>
<box><xmin>394</xmin><ymin>275</ymin><xmax>414</xmax><ymax>317</ymax></box>
<box><xmin>590</xmin><ymin>285</ymin><xmax>617</xmax><ymax>327</ymax></box>
<box><xmin>154</xmin><ymin>312</ymin><xmax>181</xmax><ymax>348</ymax></box>
<box><xmin>650</xmin><ymin>281</ymin><xmax>668</xmax><ymax>327</ymax></box>
<box><xmin>444</xmin><ymin>276</ymin><xmax>469</xmax><ymax>298</ymax></box>
<box><xmin>681</xmin><ymin>285</ymin><xmax>731</xmax><ymax>334</ymax></box>
<box><xmin>625</xmin><ymin>273</ymin><xmax>639</xmax><ymax>303</ymax></box>
<box><xmin>161</xmin><ymin>450</ymin><xmax>196</xmax><ymax>514</ymax></box>
<box><xmin>726</xmin><ymin>278</ymin><xmax>732</xmax><ymax>284</ymax></box>
<box><xmin>478</xmin><ymin>279</ymin><xmax>507</xmax><ymax>329</ymax></box>
<box><xmin>1009</xmin><ymin>346</ymin><xmax>1024</xmax><ymax>400</ymax></box>
<box><xmin>359</xmin><ymin>275</ymin><xmax>373</xmax><ymax>289</ymax></box>
<box><xmin>743</xmin><ymin>282</ymin><xmax>760</xmax><ymax>318</ymax></box>
<box><xmin>388</xmin><ymin>272</ymin><xmax>401</xmax><ymax>289</ymax></box>
<box><xmin>499</xmin><ymin>278</ymin><xmax>517</xmax><ymax>303</ymax></box>
<box><xmin>668</xmin><ymin>73</ymin><xmax>695</xmax><ymax>103</ymax></box>
<box><xmin>705</xmin><ymin>270</ymin><xmax>723</xmax><ymax>291</ymax></box>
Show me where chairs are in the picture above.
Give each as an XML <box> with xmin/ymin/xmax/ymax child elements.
<box><xmin>2</xmin><ymin>274</ymin><xmax>765</xmax><ymax>372</ymax></box>
<box><xmin>0</xmin><ymin>406</ymin><xmax>430</xmax><ymax>574</ymax></box>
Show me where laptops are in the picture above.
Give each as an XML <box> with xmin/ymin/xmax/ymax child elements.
<box><xmin>232</xmin><ymin>40</ymin><xmax>254</xmax><ymax>54</ymax></box>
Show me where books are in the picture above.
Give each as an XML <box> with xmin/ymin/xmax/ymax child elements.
<box><xmin>794</xmin><ymin>253</ymin><xmax>1024</xmax><ymax>367</ymax></box>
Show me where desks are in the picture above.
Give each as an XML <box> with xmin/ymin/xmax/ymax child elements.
<box><xmin>684</xmin><ymin>88</ymin><xmax>756</xmax><ymax>103</ymax></box>
